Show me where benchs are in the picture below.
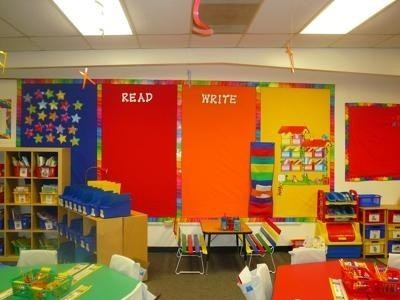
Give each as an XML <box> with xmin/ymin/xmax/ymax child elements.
<box><xmin>171</xmin><ymin>213</ymin><xmax>208</xmax><ymax>277</ymax></box>
<box><xmin>237</xmin><ymin>217</ymin><xmax>281</xmax><ymax>273</ymax></box>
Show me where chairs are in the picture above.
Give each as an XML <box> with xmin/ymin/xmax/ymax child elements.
<box><xmin>16</xmin><ymin>248</ymin><xmax>58</xmax><ymax>266</ymax></box>
<box><xmin>110</xmin><ymin>254</ymin><xmax>145</xmax><ymax>284</ymax></box>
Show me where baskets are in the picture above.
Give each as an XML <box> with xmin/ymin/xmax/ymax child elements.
<box><xmin>38</xmin><ymin>216</ymin><xmax>58</xmax><ymax>230</ymax></box>
<box><xmin>359</xmin><ymin>194</ymin><xmax>382</xmax><ymax>207</ymax></box>
<box><xmin>362</xmin><ymin>240</ymin><xmax>385</xmax><ymax>254</ymax></box>
<box><xmin>360</xmin><ymin>225</ymin><xmax>385</xmax><ymax>239</ymax></box>
<box><xmin>338</xmin><ymin>258</ymin><xmax>400</xmax><ymax>300</ymax></box>
<box><xmin>33</xmin><ymin>164</ymin><xmax>58</xmax><ymax>177</ymax></box>
<box><xmin>388</xmin><ymin>210</ymin><xmax>400</xmax><ymax>224</ymax></box>
<box><xmin>11</xmin><ymin>238</ymin><xmax>58</xmax><ymax>256</ymax></box>
<box><xmin>39</xmin><ymin>192</ymin><xmax>57</xmax><ymax>204</ymax></box>
<box><xmin>388</xmin><ymin>241</ymin><xmax>400</xmax><ymax>252</ymax></box>
<box><xmin>387</xmin><ymin>225</ymin><xmax>400</xmax><ymax>240</ymax></box>
<box><xmin>8</xmin><ymin>213</ymin><xmax>31</xmax><ymax>230</ymax></box>
<box><xmin>0</xmin><ymin>162</ymin><xmax>4</xmax><ymax>256</ymax></box>
<box><xmin>12</xmin><ymin>191</ymin><xmax>31</xmax><ymax>203</ymax></box>
<box><xmin>360</xmin><ymin>211</ymin><xmax>384</xmax><ymax>223</ymax></box>
<box><xmin>12</xmin><ymin>165</ymin><xmax>30</xmax><ymax>177</ymax></box>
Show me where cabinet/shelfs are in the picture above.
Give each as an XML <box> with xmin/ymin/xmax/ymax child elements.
<box><xmin>317</xmin><ymin>190</ymin><xmax>400</xmax><ymax>264</ymax></box>
<box><xmin>1</xmin><ymin>146</ymin><xmax>71</xmax><ymax>263</ymax></box>
<box><xmin>55</xmin><ymin>204</ymin><xmax>149</xmax><ymax>282</ymax></box>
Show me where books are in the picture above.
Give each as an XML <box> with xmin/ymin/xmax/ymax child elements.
<box><xmin>10</xmin><ymin>206</ymin><xmax>25</xmax><ymax>219</ymax></box>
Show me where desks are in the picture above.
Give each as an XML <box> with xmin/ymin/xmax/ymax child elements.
<box><xmin>271</xmin><ymin>257</ymin><xmax>400</xmax><ymax>300</ymax></box>
<box><xmin>1</xmin><ymin>264</ymin><xmax>139</xmax><ymax>300</ymax></box>
<box><xmin>199</xmin><ymin>218</ymin><xmax>253</xmax><ymax>276</ymax></box>
